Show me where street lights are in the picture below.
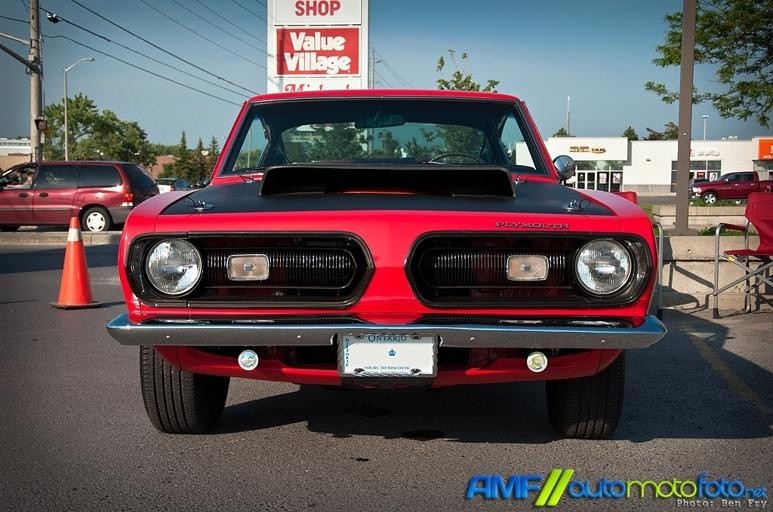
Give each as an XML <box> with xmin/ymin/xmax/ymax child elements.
<box><xmin>64</xmin><ymin>56</ymin><xmax>95</xmax><ymax>162</ymax></box>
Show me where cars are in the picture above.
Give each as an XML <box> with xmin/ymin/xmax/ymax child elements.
<box><xmin>156</xmin><ymin>177</ymin><xmax>188</xmax><ymax>194</ymax></box>
<box><xmin>105</xmin><ymin>92</ymin><xmax>667</xmax><ymax>439</ymax></box>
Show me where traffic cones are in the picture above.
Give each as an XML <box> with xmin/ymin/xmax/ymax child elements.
<box><xmin>49</xmin><ymin>217</ymin><xmax>102</xmax><ymax>309</ymax></box>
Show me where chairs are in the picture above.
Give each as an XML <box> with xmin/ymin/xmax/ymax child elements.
<box><xmin>712</xmin><ymin>193</ymin><xmax>773</xmax><ymax>319</ymax></box>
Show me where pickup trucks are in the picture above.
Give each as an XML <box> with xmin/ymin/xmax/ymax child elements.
<box><xmin>691</xmin><ymin>171</ymin><xmax>772</xmax><ymax>205</ymax></box>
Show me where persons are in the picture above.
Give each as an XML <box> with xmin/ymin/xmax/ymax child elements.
<box><xmin>7</xmin><ymin>173</ymin><xmax>34</xmax><ymax>188</ymax></box>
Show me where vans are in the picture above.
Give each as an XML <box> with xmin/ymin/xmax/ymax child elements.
<box><xmin>1</xmin><ymin>161</ymin><xmax>158</xmax><ymax>233</ymax></box>
